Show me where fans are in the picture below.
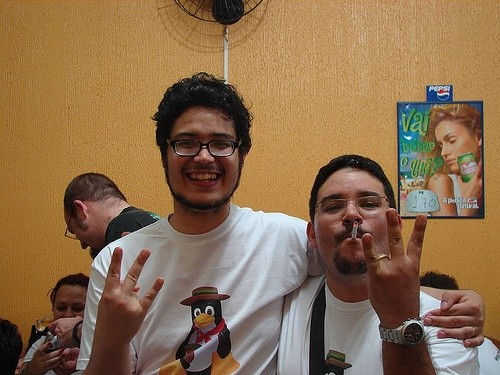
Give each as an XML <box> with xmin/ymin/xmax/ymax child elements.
<box><xmin>174</xmin><ymin>0</ymin><xmax>263</xmax><ymax>26</ymax></box>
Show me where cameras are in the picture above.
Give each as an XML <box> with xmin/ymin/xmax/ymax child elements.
<box><xmin>43</xmin><ymin>332</ymin><xmax>61</xmax><ymax>354</ymax></box>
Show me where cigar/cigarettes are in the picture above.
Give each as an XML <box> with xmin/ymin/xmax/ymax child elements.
<box><xmin>352</xmin><ymin>221</ymin><xmax>358</xmax><ymax>239</ymax></box>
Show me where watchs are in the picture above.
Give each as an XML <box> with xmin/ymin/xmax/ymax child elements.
<box><xmin>379</xmin><ymin>318</ymin><xmax>425</xmax><ymax>346</ymax></box>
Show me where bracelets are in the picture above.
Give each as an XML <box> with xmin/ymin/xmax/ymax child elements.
<box><xmin>72</xmin><ymin>321</ymin><xmax>83</xmax><ymax>347</ymax></box>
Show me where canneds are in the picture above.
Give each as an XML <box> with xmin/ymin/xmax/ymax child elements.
<box><xmin>457</xmin><ymin>152</ymin><xmax>477</xmax><ymax>182</ymax></box>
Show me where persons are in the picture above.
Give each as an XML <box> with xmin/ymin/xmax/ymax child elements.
<box><xmin>123</xmin><ymin>156</ymin><xmax>479</xmax><ymax>375</ymax></box>
<box><xmin>419</xmin><ymin>272</ymin><xmax>500</xmax><ymax>375</ymax></box>
<box><xmin>0</xmin><ymin>173</ymin><xmax>162</xmax><ymax>375</ymax></box>
<box><xmin>76</xmin><ymin>72</ymin><xmax>486</xmax><ymax>375</ymax></box>
<box><xmin>400</xmin><ymin>104</ymin><xmax>484</xmax><ymax>216</ymax></box>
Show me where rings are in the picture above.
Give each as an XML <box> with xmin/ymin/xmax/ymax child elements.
<box><xmin>376</xmin><ymin>254</ymin><xmax>391</xmax><ymax>261</ymax></box>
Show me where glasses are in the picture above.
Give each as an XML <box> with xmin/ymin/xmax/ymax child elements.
<box><xmin>65</xmin><ymin>203</ymin><xmax>80</xmax><ymax>239</ymax></box>
<box><xmin>312</xmin><ymin>195</ymin><xmax>389</xmax><ymax>216</ymax></box>
<box><xmin>165</xmin><ymin>136</ymin><xmax>247</xmax><ymax>157</ymax></box>
